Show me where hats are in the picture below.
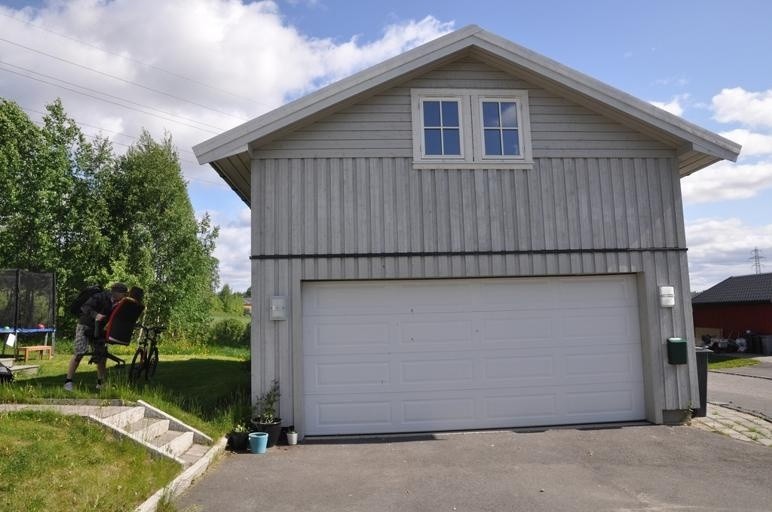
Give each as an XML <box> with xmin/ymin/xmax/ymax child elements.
<box><xmin>111</xmin><ymin>283</ymin><xmax>129</xmax><ymax>294</ymax></box>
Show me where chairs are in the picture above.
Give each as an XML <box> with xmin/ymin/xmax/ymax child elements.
<box><xmin>77</xmin><ymin>296</ymin><xmax>145</xmax><ymax>372</ymax></box>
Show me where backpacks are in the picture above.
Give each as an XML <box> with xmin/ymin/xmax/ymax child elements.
<box><xmin>69</xmin><ymin>285</ymin><xmax>108</xmax><ymax>319</ymax></box>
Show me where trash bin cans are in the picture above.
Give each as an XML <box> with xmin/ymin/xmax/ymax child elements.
<box><xmin>694</xmin><ymin>345</ymin><xmax>714</xmax><ymax>417</ymax></box>
<box><xmin>752</xmin><ymin>335</ymin><xmax>772</xmax><ymax>355</ymax></box>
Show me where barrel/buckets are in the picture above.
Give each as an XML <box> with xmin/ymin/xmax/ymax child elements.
<box><xmin>248</xmin><ymin>432</ymin><xmax>269</xmax><ymax>454</ymax></box>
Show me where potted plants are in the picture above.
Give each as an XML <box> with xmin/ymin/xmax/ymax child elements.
<box><xmin>225</xmin><ymin>376</ymin><xmax>301</xmax><ymax>454</ymax></box>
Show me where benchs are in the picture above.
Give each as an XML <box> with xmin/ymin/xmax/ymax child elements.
<box><xmin>17</xmin><ymin>345</ymin><xmax>52</xmax><ymax>363</ymax></box>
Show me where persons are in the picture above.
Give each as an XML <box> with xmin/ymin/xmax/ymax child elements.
<box><xmin>64</xmin><ymin>283</ymin><xmax>144</xmax><ymax>392</ymax></box>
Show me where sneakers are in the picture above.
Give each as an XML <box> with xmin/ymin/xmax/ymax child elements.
<box><xmin>63</xmin><ymin>382</ymin><xmax>73</xmax><ymax>391</ymax></box>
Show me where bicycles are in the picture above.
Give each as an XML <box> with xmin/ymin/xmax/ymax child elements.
<box><xmin>126</xmin><ymin>324</ymin><xmax>169</xmax><ymax>390</ymax></box>
